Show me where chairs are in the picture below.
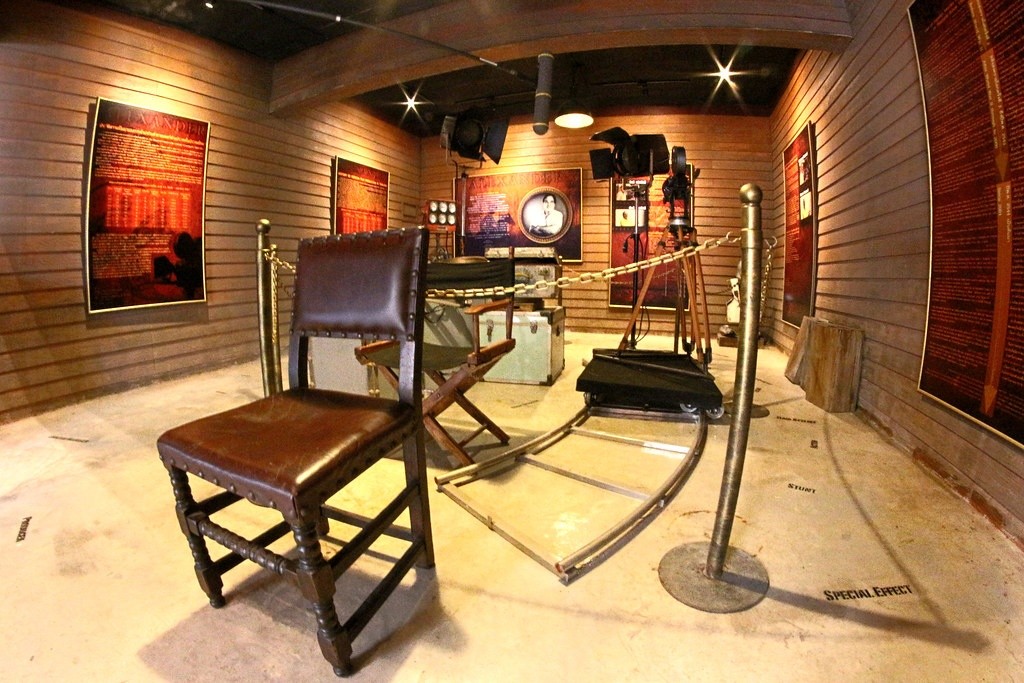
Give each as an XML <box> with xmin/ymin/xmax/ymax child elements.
<box><xmin>356</xmin><ymin>244</ymin><xmax>516</xmax><ymax>466</ymax></box>
<box><xmin>157</xmin><ymin>224</ymin><xmax>435</xmax><ymax>678</ymax></box>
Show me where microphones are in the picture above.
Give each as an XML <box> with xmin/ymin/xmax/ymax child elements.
<box><xmin>533</xmin><ymin>51</ymin><xmax>555</xmax><ymax>135</ymax></box>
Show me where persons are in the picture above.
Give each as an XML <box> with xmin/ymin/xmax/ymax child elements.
<box><xmin>528</xmin><ymin>195</ymin><xmax>562</xmax><ymax>236</ymax></box>
<box><xmin>615</xmin><ymin>185</ymin><xmax>635</xmax><ymax>226</ymax></box>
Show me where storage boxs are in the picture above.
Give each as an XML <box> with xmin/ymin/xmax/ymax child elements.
<box><xmin>479</xmin><ymin>304</ymin><xmax>565</xmax><ymax>385</ymax></box>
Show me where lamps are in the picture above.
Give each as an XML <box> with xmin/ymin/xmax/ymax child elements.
<box><xmin>552</xmin><ymin>86</ymin><xmax>594</xmax><ymax>129</ymax></box>
<box><xmin>439</xmin><ymin>106</ymin><xmax>510</xmax><ymax>170</ymax></box>
